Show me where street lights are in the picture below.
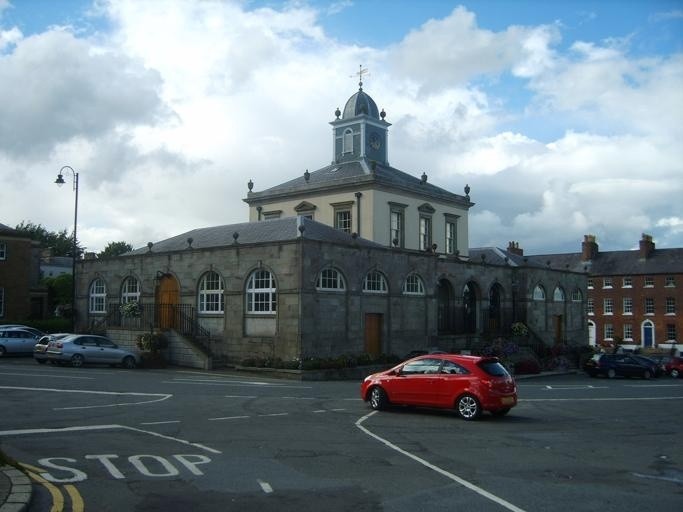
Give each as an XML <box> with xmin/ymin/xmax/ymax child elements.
<box><xmin>54</xmin><ymin>166</ymin><xmax>78</xmax><ymax>330</ymax></box>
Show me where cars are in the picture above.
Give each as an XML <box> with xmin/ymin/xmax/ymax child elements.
<box><xmin>0</xmin><ymin>325</ymin><xmax>144</xmax><ymax>369</ymax></box>
<box><xmin>360</xmin><ymin>351</ymin><xmax>517</xmax><ymax>421</ymax></box>
<box><xmin>54</xmin><ymin>304</ymin><xmax>73</xmax><ymax>318</ymax></box>
<box><xmin>587</xmin><ymin>353</ymin><xmax>683</xmax><ymax>378</ymax></box>
